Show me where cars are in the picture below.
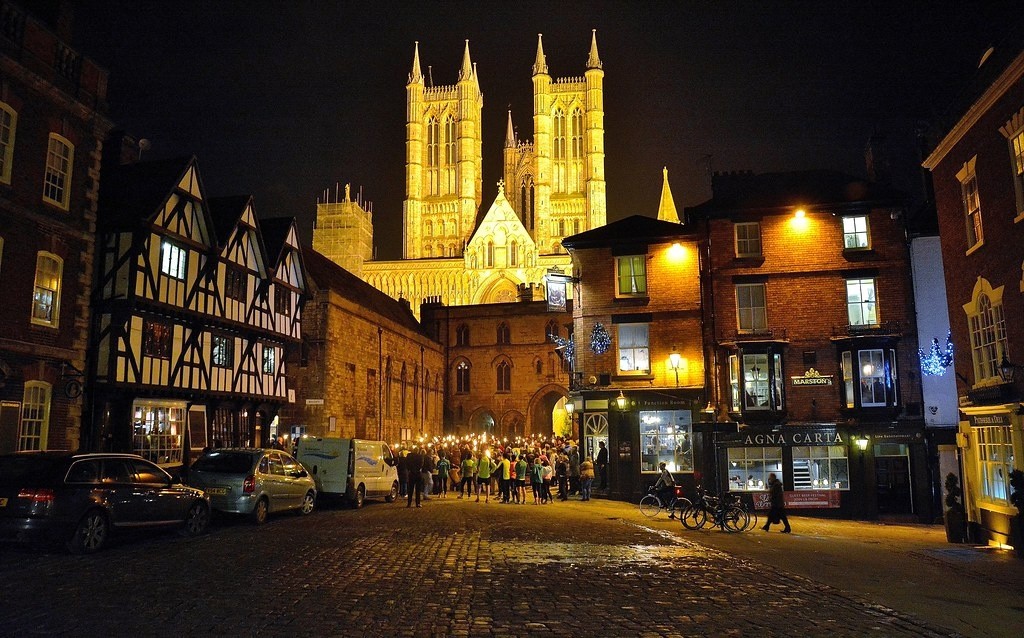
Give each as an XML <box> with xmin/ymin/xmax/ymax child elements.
<box><xmin>0</xmin><ymin>450</ymin><xmax>212</xmax><ymax>555</ymax></box>
<box><xmin>188</xmin><ymin>446</ymin><xmax>318</xmax><ymax>523</ymax></box>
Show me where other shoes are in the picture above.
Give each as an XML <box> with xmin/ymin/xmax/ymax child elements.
<box><xmin>760</xmin><ymin>525</ymin><xmax>769</xmax><ymax>532</ymax></box>
<box><xmin>561</xmin><ymin>497</ymin><xmax>567</xmax><ymax>502</ymax></box>
<box><xmin>493</xmin><ymin>496</ymin><xmax>501</xmax><ymax>501</ymax></box>
<box><xmin>474</xmin><ymin>498</ymin><xmax>479</xmax><ymax>503</ymax></box>
<box><xmin>542</xmin><ymin>500</ymin><xmax>548</xmax><ymax>504</ymax></box>
<box><xmin>579</xmin><ymin>498</ymin><xmax>590</xmax><ymax>501</ymax></box>
<box><xmin>467</xmin><ymin>494</ymin><xmax>471</xmax><ymax>499</ymax></box>
<box><xmin>522</xmin><ymin>500</ymin><xmax>526</xmax><ymax>504</ymax></box>
<box><xmin>660</xmin><ymin>503</ymin><xmax>667</xmax><ymax>509</ymax></box>
<box><xmin>551</xmin><ymin>500</ymin><xmax>555</xmax><ymax>504</ymax></box>
<box><xmin>405</xmin><ymin>502</ymin><xmax>412</xmax><ymax>508</ymax></box>
<box><xmin>443</xmin><ymin>495</ymin><xmax>447</xmax><ymax>498</ymax></box>
<box><xmin>423</xmin><ymin>497</ymin><xmax>432</xmax><ymax>500</ymax></box>
<box><xmin>457</xmin><ymin>495</ymin><xmax>463</xmax><ymax>499</ymax></box>
<box><xmin>438</xmin><ymin>495</ymin><xmax>441</xmax><ymax>499</ymax></box>
<box><xmin>490</xmin><ymin>493</ymin><xmax>495</xmax><ymax>495</ymax></box>
<box><xmin>515</xmin><ymin>500</ymin><xmax>520</xmax><ymax>505</ymax></box>
<box><xmin>500</xmin><ymin>499</ymin><xmax>510</xmax><ymax>504</ymax></box>
<box><xmin>510</xmin><ymin>499</ymin><xmax>516</xmax><ymax>503</ymax></box>
<box><xmin>485</xmin><ymin>498</ymin><xmax>490</xmax><ymax>504</ymax></box>
<box><xmin>668</xmin><ymin>514</ymin><xmax>675</xmax><ymax>520</ymax></box>
<box><xmin>416</xmin><ymin>504</ymin><xmax>422</xmax><ymax>508</ymax></box>
<box><xmin>531</xmin><ymin>501</ymin><xmax>541</xmax><ymax>506</ymax></box>
<box><xmin>398</xmin><ymin>494</ymin><xmax>408</xmax><ymax>500</ymax></box>
<box><xmin>780</xmin><ymin>527</ymin><xmax>791</xmax><ymax>533</ymax></box>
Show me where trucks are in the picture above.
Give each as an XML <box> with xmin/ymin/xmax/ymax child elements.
<box><xmin>297</xmin><ymin>437</ymin><xmax>401</xmax><ymax>507</ymax></box>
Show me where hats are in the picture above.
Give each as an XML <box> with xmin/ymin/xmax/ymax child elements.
<box><xmin>659</xmin><ymin>462</ymin><xmax>666</xmax><ymax>467</ymax></box>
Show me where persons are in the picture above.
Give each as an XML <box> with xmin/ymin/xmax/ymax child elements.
<box><xmin>580</xmin><ymin>457</ymin><xmax>594</xmax><ymax>501</ymax></box>
<box><xmin>436</xmin><ymin>452</ymin><xmax>450</xmax><ymax>498</ymax></box>
<box><xmin>650</xmin><ymin>462</ymin><xmax>676</xmax><ymax>518</ymax></box>
<box><xmin>682</xmin><ymin>433</ymin><xmax>690</xmax><ymax>452</ymax></box>
<box><xmin>762</xmin><ymin>473</ymin><xmax>791</xmax><ymax>533</ymax></box>
<box><xmin>514</xmin><ymin>454</ymin><xmax>527</xmax><ymax>504</ymax></box>
<box><xmin>457</xmin><ymin>454</ymin><xmax>476</xmax><ymax>499</ymax></box>
<box><xmin>267</xmin><ymin>434</ymin><xmax>299</xmax><ymax>457</ymax></box>
<box><xmin>404</xmin><ymin>446</ymin><xmax>423</xmax><ymax>508</ymax></box>
<box><xmin>388</xmin><ymin>435</ymin><xmax>578</xmax><ymax>505</ymax></box>
<box><xmin>473</xmin><ymin>452</ymin><xmax>496</xmax><ymax>503</ymax></box>
<box><xmin>595</xmin><ymin>441</ymin><xmax>607</xmax><ymax>490</ymax></box>
<box><xmin>133</xmin><ymin>427</ymin><xmax>170</xmax><ymax>463</ymax></box>
<box><xmin>861</xmin><ymin>378</ymin><xmax>884</xmax><ymax>402</ymax></box>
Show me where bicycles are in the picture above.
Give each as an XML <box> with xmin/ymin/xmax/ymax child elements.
<box><xmin>640</xmin><ymin>486</ymin><xmax>693</xmax><ymax>520</ymax></box>
<box><xmin>680</xmin><ymin>484</ymin><xmax>757</xmax><ymax>533</ymax></box>
